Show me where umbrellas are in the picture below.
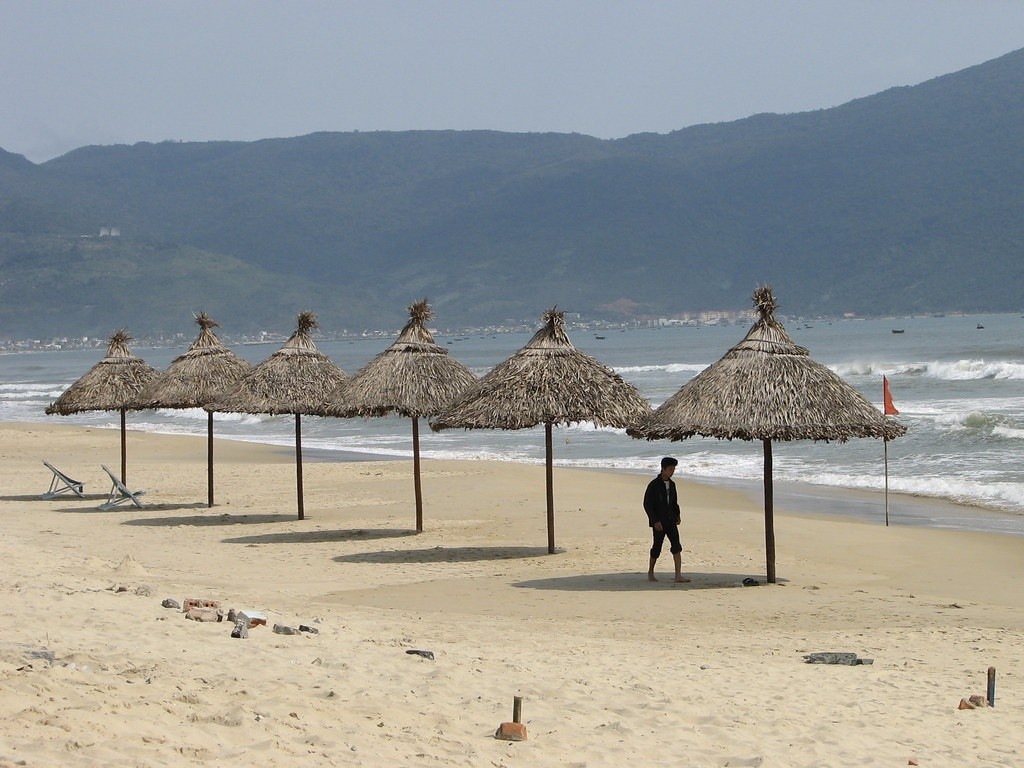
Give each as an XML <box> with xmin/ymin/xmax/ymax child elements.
<box><xmin>628</xmin><ymin>281</ymin><xmax>908</xmax><ymax>578</ymax></box>
<box><xmin>203</xmin><ymin>311</ymin><xmax>389</xmax><ymax>516</ymax></box>
<box><xmin>318</xmin><ymin>299</ymin><xmax>476</xmax><ymax>533</ymax></box>
<box><xmin>431</xmin><ymin>306</ymin><xmax>657</xmax><ymax>552</ymax></box>
<box><xmin>125</xmin><ymin>313</ymin><xmax>252</xmax><ymax>508</ymax></box>
<box><xmin>45</xmin><ymin>333</ymin><xmax>164</xmax><ymax>496</ymax></box>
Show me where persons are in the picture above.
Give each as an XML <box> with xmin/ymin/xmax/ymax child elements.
<box><xmin>643</xmin><ymin>457</ymin><xmax>690</xmax><ymax>582</ymax></box>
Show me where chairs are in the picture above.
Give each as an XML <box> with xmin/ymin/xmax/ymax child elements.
<box><xmin>98</xmin><ymin>463</ymin><xmax>146</xmax><ymax>510</ymax></box>
<box><xmin>41</xmin><ymin>459</ymin><xmax>84</xmax><ymax>498</ymax></box>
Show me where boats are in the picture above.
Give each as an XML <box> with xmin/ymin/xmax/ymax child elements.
<box><xmin>893</xmin><ymin>330</ymin><xmax>905</xmax><ymax>334</ymax></box>
<box><xmin>447</xmin><ymin>336</ymin><xmax>496</xmax><ymax>343</ymax></box>
<box><xmin>596</xmin><ymin>336</ymin><xmax>606</xmax><ymax>338</ymax></box>
